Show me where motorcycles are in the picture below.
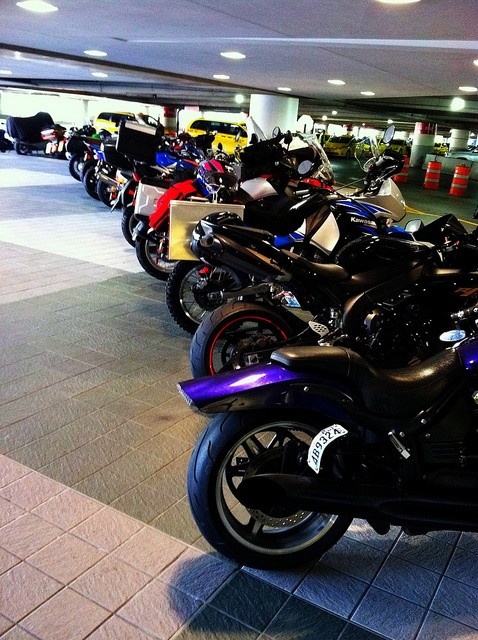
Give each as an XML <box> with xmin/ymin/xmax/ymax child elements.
<box><xmin>188</xmin><ymin>207</ymin><xmax>477</xmax><ymax>380</ymax></box>
<box><xmin>168</xmin><ymin>151</ymin><xmax>407</xmax><ymax>334</ymax></box>
<box><xmin>176</xmin><ymin>303</ymin><xmax>478</xmax><ymax>571</ymax></box>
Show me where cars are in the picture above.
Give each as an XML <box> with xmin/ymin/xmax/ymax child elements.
<box><xmin>315</xmin><ymin>133</ymin><xmax>333</xmax><ymax>147</ymax></box>
<box><xmin>96</xmin><ymin>111</ymin><xmax>177</xmax><ymax>140</ymax></box>
<box><xmin>444</xmin><ymin>145</ymin><xmax>478</xmax><ymax>162</ymax></box>
<box><xmin>433</xmin><ymin>143</ymin><xmax>448</xmax><ymax>155</ymax></box>
<box><xmin>322</xmin><ymin>135</ymin><xmax>406</xmax><ymax>158</ymax></box>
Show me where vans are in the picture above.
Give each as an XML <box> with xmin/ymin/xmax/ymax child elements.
<box><xmin>185</xmin><ymin>117</ymin><xmax>247</xmax><ymax>154</ymax></box>
